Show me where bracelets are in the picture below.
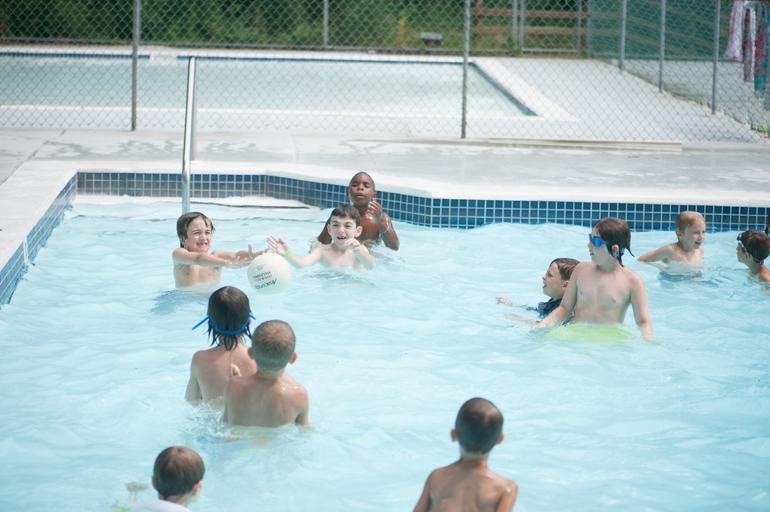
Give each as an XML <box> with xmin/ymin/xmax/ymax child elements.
<box><xmin>381</xmin><ymin>225</ymin><xmax>389</xmax><ymax>234</ymax></box>
<box><xmin>283</xmin><ymin>249</ymin><xmax>292</xmax><ymax>257</ymax></box>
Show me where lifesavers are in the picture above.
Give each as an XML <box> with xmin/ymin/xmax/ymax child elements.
<box><xmin>547</xmin><ymin>323</ymin><xmax>640</xmax><ymax>340</ymax></box>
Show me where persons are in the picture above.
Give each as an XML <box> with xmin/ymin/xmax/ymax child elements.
<box><xmin>411</xmin><ymin>395</ymin><xmax>521</xmax><ymax>511</ymax></box>
<box><xmin>315</xmin><ymin>172</ymin><xmax>401</xmax><ymax>253</ymax></box>
<box><xmin>493</xmin><ymin>256</ymin><xmax>581</xmax><ymax>331</ymax></box>
<box><xmin>531</xmin><ymin>217</ymin><xmax>652</xmax><ymax>339</ymax></box>
<box><xmin>222</xmin><ymin>317</ymin><xmax>310</xmax><ymax>427</ymax></box>
<box><xmin>183</xmin><ymin>284</ymin><xmax>259</xmax><ymax>407</ymax></box>
<box><xmin>266</xmin><ymin>202</ymin><xmax>374</xmax><ymax>270</ymax></box>
<box><xmin>172</xmin><ymin>211</ymin><xmax>269</xmax><ymax>291</ymax></box>
<box><xmin>130</xmin><ymin>445</ymin><xmax>206</xmax><ymax>512</ymax></box>
<box><xmin>735</xmin><ymin>230</ymin><xmax>770</xmax><ymax>284</ymax></box>
<box><xmin>635</xmin><ymin>209</ymin><xmax>707</xmax><ymax>285</ymax></box>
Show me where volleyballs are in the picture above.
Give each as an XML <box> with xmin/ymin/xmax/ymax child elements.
<box><xmin>248</xmin><ymin>253</ymin><xmax>290</xmax><ymax>294</ymax></box>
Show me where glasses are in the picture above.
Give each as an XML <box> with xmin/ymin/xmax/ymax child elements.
<box><xmin>588</xmin><ymin>232</ymin><xmax>610</xmax><ymax>248</ymax></box>
<box><xmin>737</xmin><ymin>233</ymin><xmax>747</xmax><ymax>253</ymax></box>
